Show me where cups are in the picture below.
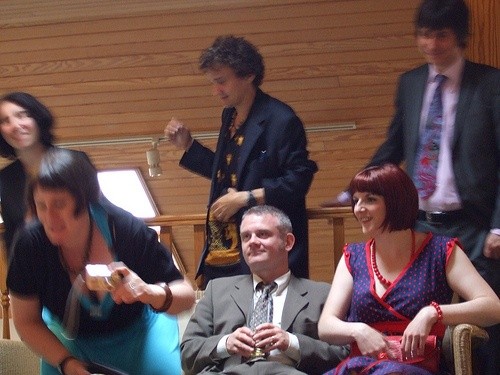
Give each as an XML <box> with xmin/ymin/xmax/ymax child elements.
<box><xmin>246</xmin><ymin>334</ymin><xmax>271</xmax><ymax>359</ymax></box>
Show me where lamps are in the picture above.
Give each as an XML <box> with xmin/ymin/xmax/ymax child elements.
<box><xmin>145</xmin><ymin>137</ymin><xmax>163</xmax><ymax>177</ymax></box>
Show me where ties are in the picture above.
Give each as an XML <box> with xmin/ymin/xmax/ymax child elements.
<box><xmin>246</xmin><ymin>281</ymin><xmax>277</xmax><ymax>364</ymax></box>
<box><xmin>413</xmin><ymin>73</ymin><xmax>448</xmax><ymax>201</ymax></box>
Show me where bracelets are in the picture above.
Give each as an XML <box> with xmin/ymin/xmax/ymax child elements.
<box><xmin>150</xmin><ymin>281</ymin><xmax>173</xmax><ymax>314</ymax></box>
<box><xmin>430</xmin><ymin>301</ymin><xmax>444</xmax><ymax>324</ymax></box>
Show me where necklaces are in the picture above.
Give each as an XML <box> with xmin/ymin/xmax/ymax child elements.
<box><xmin>58</xmin><ymin>211</ymin><xmax>101</xmax><ymax>309</ymax></box>
<box><xmin>229</xmin><ymin>111</ymin><xmax>245</xmax><ymax>132</ymax></box>
<box><xmin>371</xmin><ymin>227</ymin><xmax>416</xmax><ymax>287</ymax></box>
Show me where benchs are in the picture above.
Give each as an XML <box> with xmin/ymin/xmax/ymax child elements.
<box><xmin>0</xmin><ymin>290</ymin><xmax>489</xmax><ymax>375</ymax></box>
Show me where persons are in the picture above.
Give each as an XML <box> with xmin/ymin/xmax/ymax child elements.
<box><xmin>318</xmin><ymin>164</ymin><xmax>500</xmax><ymax>375</ymax></box>
<box><xmin>0</xmin><ymin>92</ymin><xmax>59</xmax><ymax>310</ymax></box>
<box><xmin>163</xmin><ymin>33</ymin><xmax>318</xmax><ymax>290</ymax></box>
<box><xmin>9</xmin><ymin>148</ymin><xmax>195</xmax><ymax>375</ymax></box>
<box><xmin>322</xmin><ymin>0</ymin><xmax>500</xmax><ymax>300</ymax></box>
<box><xmin>179</xmin><ymin>205</ymin><xmax>352</xmax><ymax>375</ymax></box>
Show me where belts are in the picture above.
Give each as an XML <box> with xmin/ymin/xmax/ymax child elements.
<box><xmin>417</xmin><ymin>209</ymin><xmax>469</xmax><ymax>224</ymax></box>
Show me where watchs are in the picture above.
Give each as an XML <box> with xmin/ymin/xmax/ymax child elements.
<box><xmin>247</xmin><ymin>190</ymin><xmax>258</xmax><ymax>207</ymax></box>
<box><xmin>56</xmin><ymin>356</ymin><xmax>75</xmax><ymax>375</ymax></box>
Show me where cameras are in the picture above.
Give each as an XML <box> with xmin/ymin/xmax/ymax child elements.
<box><xmin>85</xmin><ymin>264</ymin><xmax>121</xmax><ymax>293</ymax></box>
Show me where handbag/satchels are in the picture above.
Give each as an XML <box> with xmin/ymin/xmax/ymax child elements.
<box><xmin>350</xmin><ymin>335</ymin><xmax>440</xmax><ymax>375</ymax></box>
<box><xmin>205</xmin><ymin>210</ymin><xmax>241</xmax><ymax>267</ymax></box>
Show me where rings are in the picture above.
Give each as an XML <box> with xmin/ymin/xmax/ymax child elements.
<box><xmin>272</xmin><ymin>336</ymin><xmax>276</xmax><ymax>343</ymax></box>
